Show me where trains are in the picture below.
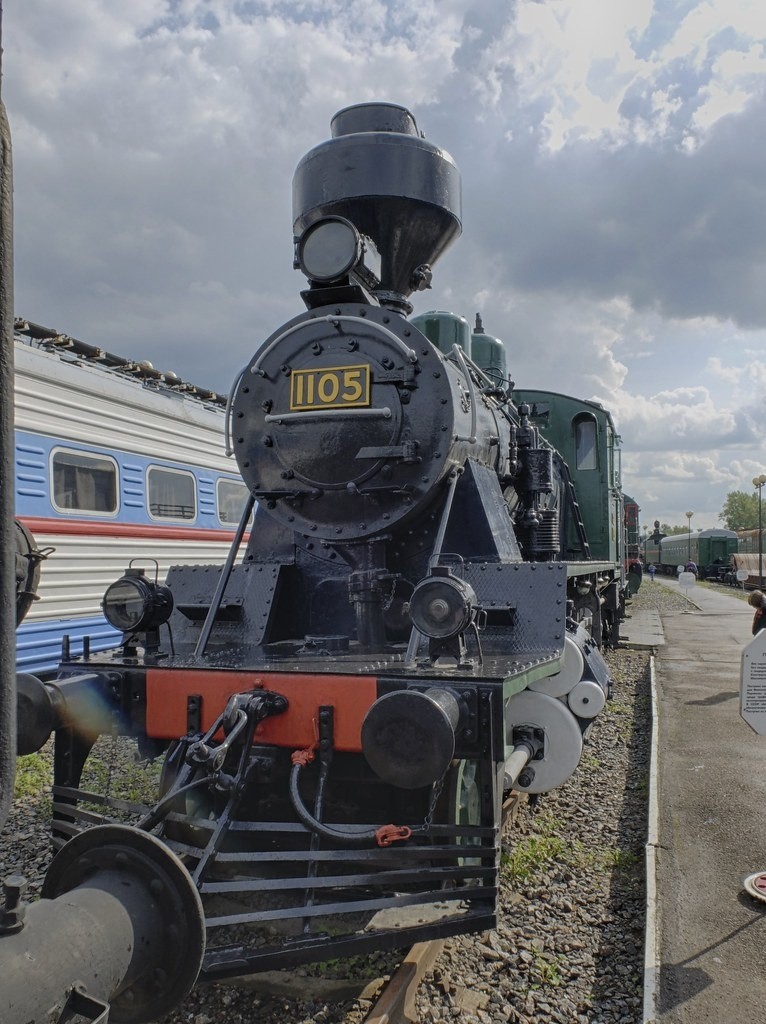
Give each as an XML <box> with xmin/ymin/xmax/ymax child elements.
<box><xmin>11</xmin><ymin>318</ymin><xmax>256</xmax><ymax>679</ymax></box>
<box><xmin>0</xmin><ymin>104</ymin><xmax>644</xmax><ymax>1024</ymax></box>
<box><xmin>644</xmin><ymin>527</ymin><xmax>766</xmax><ymax>590</ymax></box>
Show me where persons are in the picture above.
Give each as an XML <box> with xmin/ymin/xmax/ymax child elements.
<box><xmin>648</xmin><ymin>563</ymin><xmax>657</xmax><ymax>581</ymax></box>
<box><xmin>747</xmin><ymin>590</ymin><xmax>766</xmax><ymax>635</ymax></box>
<box><xmin>686</xmin><ymin>558</ymin><xmax>698</xmax><ymax>579</ymax></box>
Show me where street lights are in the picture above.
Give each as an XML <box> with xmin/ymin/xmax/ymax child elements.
<box><xmin>642</xmin><ymin>524</ymin><xmax>647</xmax><ymax>566</ymax></box>
<box><xmin>752</xmin><ymin>474</ymin><xmax>766</xmax><ymax>593</ymax></box>
<box><xmin>686</xmin><ymin>510</ymin><xmax>693</xmax><ymax>571</ymax></box>
